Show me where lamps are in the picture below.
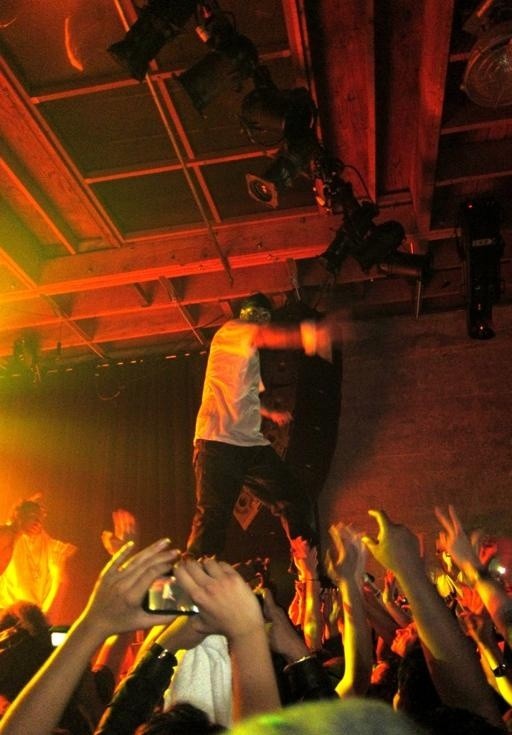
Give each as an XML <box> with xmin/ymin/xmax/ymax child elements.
<box><xmin>171</xmin><ymin>30</ymin><xmax>258</xmax><ymax>122</ymax></box>
<box><xmin>106</xmin><ymin>1</ymin><xmax>197</xmax><ymax>83</ymax></box>
<box><xmin>239</xmin><ymin>86</ymin><xmax>319</xmax><ymax>150</ymax></box>
<box><xmin>377</xmin><ymin>249</ymin><xmax>435</xmax><ymax>284</ymax></box>
<box><xmin>244</xmin><ymin>134</ymin><xmax>318</xmax><ymax>209</ymax></box>
<box><xmin>319</xmin><ymin>199</ymin><xmax>381</xmax><ymax>276</ymax></box>
<box><xmin>352</xmin><ymin>218</ymin><xmax>406</xmax><ymax>270</ymax></box>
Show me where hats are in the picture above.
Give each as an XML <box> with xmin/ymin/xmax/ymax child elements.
<box><xmin>240</xmin><ymin>291</ymin><xmax>273</xmax><ymax>311</ymax></box>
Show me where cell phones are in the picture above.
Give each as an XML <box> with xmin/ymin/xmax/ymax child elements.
<box><xmin>142</xmin><ymin>570</ymin><xmax>199</xmax><ymax>614</ymax></box>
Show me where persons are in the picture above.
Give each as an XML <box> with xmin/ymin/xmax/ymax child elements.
<box><xmin>0</xmin><ymin>507</ymin><xmax>511</xmax><ymax>733</ymax></box>
<box><xmin>1</xmin><ymin>488</ymin><xmax>44</xmax><ymax>578</ymax></box>
<box><xmin>183</xmin><ymin>290</ymin><xmax>353</xmax><ymax>564</ymax></box>
